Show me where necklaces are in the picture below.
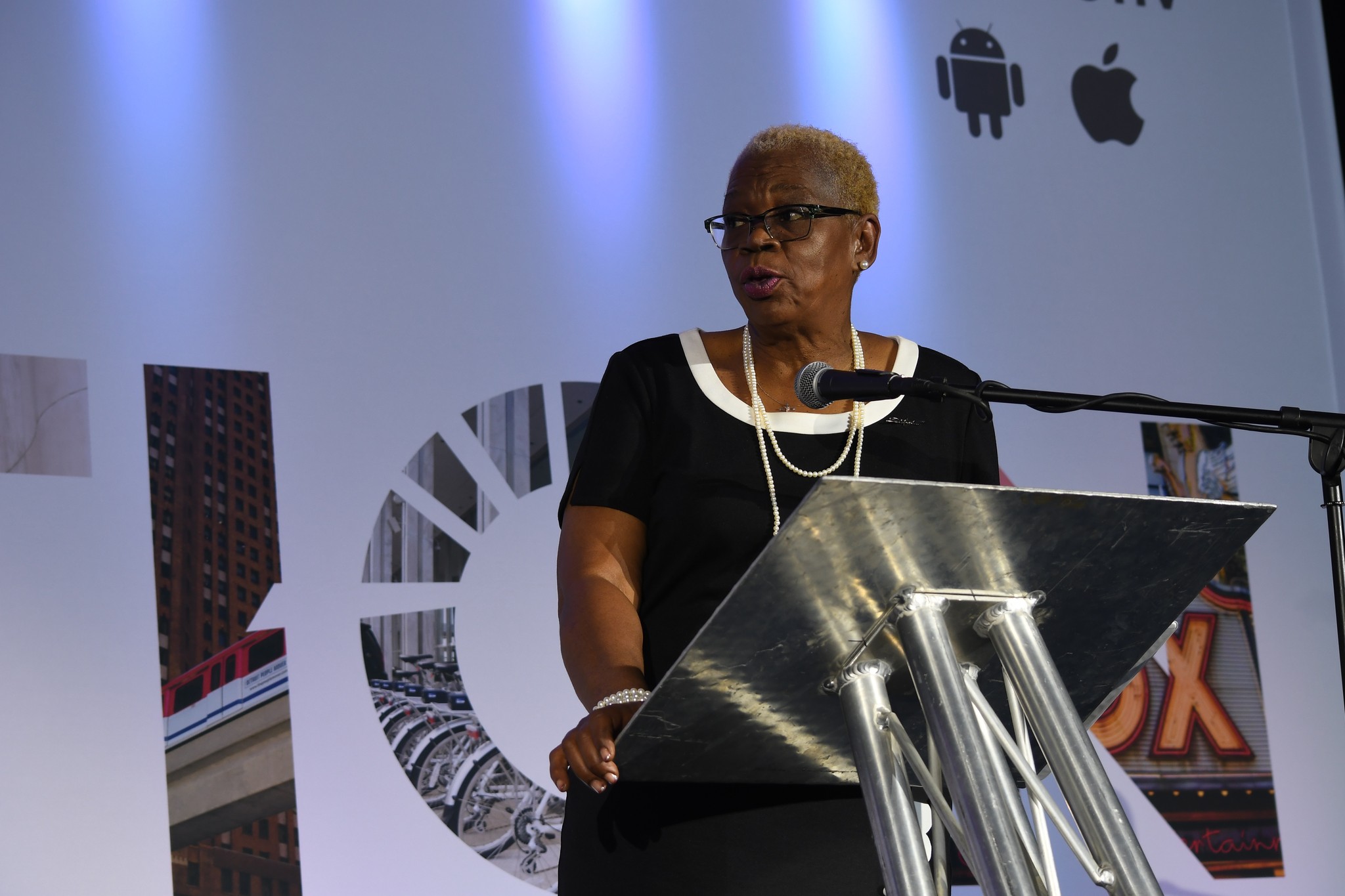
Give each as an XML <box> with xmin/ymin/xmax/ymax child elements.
<box><xmin>744</xmin><ymin>319</ymin><xmax>864</xmax><ymax>536</ymax></box>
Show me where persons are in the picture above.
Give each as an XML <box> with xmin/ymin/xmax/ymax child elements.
<box><xmin>549</xmin><ymin>122</ymin><xmax>1000</xmax><ymax>794</ymax></box>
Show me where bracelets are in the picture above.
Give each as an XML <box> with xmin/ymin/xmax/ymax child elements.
<box><xmin>591</xmin><ymin>689</ymin><xmax>652</xmax><ymax>712</ymax></box>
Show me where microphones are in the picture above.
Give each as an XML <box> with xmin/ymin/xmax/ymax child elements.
<box><xmin>793</xmin><ymin>362</ymin><xmax>930</xmax><ymax>410</ymax></box>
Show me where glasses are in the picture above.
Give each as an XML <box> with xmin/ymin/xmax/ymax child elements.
<box><xmin>701</xmin><ymin>207</ymin><xmax>867</xmax><ymax>256</ymax></box>
